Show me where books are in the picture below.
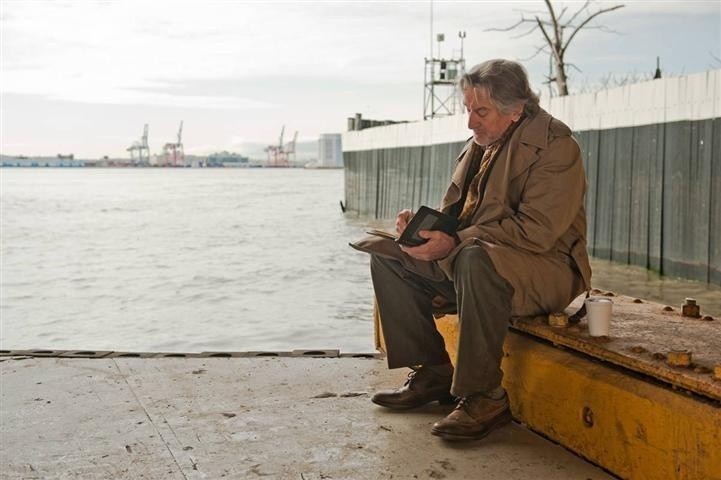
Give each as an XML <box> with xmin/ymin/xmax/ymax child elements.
<box><xmin>367</xmin><ymin>206</ymin><xmax>459</xmax><ymax>249</ymax></box>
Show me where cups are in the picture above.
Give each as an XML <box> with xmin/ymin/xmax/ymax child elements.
<box><xmin>585</xmin><ymin>296</ymin><xmax>614</xmax><ymax>338</ymax></box>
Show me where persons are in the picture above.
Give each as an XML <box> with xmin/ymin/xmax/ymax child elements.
<box><xmin>369</xmin><ymin>58</ymin><xmax>592</xmax><ymax>441</ymax></box>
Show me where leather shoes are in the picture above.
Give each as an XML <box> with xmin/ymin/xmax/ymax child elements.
<box><xmin>371</xmin><ymin>364</ymin><xmax>454</xmax><ymax>410</ymax></box>
<box><xmin>432</xmin><ymin>388</ymin><xmax>513</xmax><ymax>441</ymax></box>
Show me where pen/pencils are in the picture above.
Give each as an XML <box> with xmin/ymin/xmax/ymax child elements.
<box><xmin>409</xmin><ymin>208</ymin><xmax>413</xmax><ymax>216</ymax></box>
